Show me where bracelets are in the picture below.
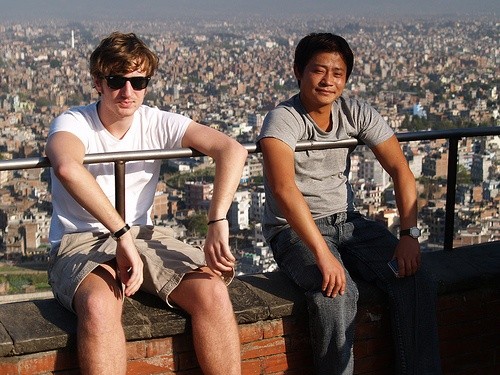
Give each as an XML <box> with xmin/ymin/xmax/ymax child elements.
<box><xmin>207</xmin><ymin>218</ymin><xmax>227</xmax><ymax>225</ymax></box>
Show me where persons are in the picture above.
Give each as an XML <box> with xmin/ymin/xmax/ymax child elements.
<box><xmin>47</xmin><ymin>32</ymin><xmax>249</xmax><ymax>375</ymax></box>
<box><xmin>257</xmin><ymin>32</ymin><xmax>439</xmax><ymax>375</ymax></box>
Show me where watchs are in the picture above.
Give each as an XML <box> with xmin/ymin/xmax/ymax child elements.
<box><xmin>400</xmin><ymin>227</ymin><xmax>421</xmax><ymax>238</ymax></box>
<box><xmin>111</xmin><ymin>224</ymin><xmax>130</xmax><ymax>240</ymax></box>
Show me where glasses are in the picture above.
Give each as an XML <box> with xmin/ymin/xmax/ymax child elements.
<box><xmin>101</xmin><ymin>74</ymin><xmax>152</xmax><ymax>90</ymax></box>
